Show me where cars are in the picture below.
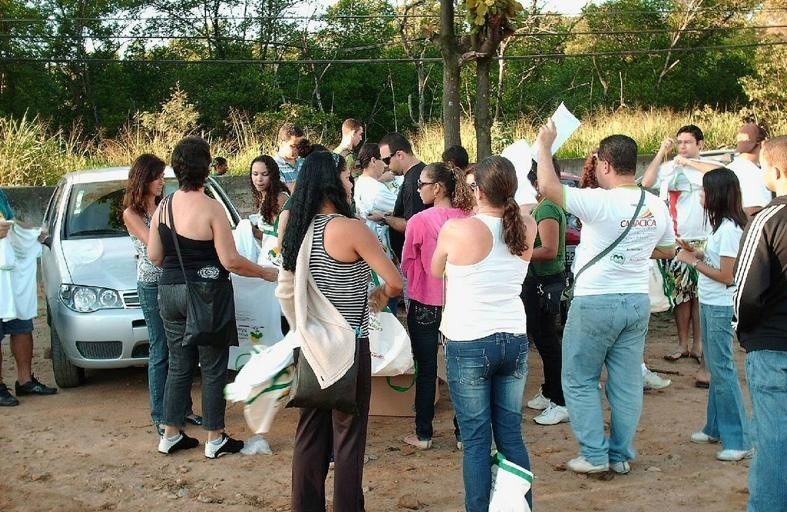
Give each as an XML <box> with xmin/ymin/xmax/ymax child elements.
<box><xmin>637</xmin><ymin>147</ymin><xmax>738</xmax><ymax>190</ymax></box>
<box><xmin>530</xmin><ymin>169</ymin><xmax>588</xmax><ymax>245</ymax></box>
<box><xmin>33</xmin><ymin>152</ymin><xmax>268</xmax><ymax>392</ymax></box>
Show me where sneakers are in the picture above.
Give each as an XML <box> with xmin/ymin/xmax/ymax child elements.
<box><xmin>185</xmin><ymin>414</ymin><xmax>202</xmax><ymax>425</ymax></box>
<box><xmin>16</xmin><ymin>372</ymin><xmax>57</xmax><ymax>396</ymax></box>
<box><xmin>400</xmin><ymin>431</ymin><xmax>433</xmax><ymax>450</ymax></box>
<box><xmin>204</xmin><ymin>432</ymin><xmax>244</xmax><ymax>459</ymax></box>
<box><xmin>690</xmin><ymin>428</ymin><xmax>754</xmax><ymax>462</ymax></box>
<box><xmin>0</xmin><ymin>383</ymin><xmax>19</xmax><ymax>406</ymax></box>
<box><xmin>531</xmin><ymin>400</ymin><xmax>571</xmax><ymax>425</ymax></box>
<box><xmin>527</xmin><ymin>389</ymin><xmax>551</xmax><ymax>410</ymax></box>
<box><xmin>568</xmin><ymin>455</ymin><xmax>631</xmax><ymax>474</ymax></box>
<box><xmin>157</xmin><ymin>425</ymin><xmax>198</xmax><ymax>454</ymax></box>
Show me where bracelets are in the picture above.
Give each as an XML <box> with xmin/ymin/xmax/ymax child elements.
<box><xmin>691</xmin><ymin>258</ymin><xmax>701</xmax><ymax>268</ymax></box>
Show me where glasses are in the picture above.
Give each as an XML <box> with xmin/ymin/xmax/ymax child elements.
<box><xmin>417</xmin><ymin>179</ymin><xmax>435</xmax><ymax>190</ymax></box>
<box><xmin>382</xmin><ymin>149</ymin><xmax>399</xmax><ymax>165</ymax></box>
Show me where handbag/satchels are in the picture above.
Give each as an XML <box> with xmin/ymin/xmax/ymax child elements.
<box><xmin>181</xmin><ymin>277</ymin><xmax>240</xmax><ymax>349</ymax></box>
<box><xmin>256</xmin><ymin>228</ymin><xmax>282</xmax><ymax>272</ymax></box>
<box><xmin>285</xmin><ymin>332</ymin><xmax>363</xmax><ymax>419</ymax></box>
<box><xmin>368</xmin><ymin>303</ymin><xmax>415</xmax><ymax>378</ymax></box>
<box><xmin>486</xmin><ymin>448</ymin><xmax>534</xmax><ymax>512</ymax></box>
<box><xmin>534</xmin><ymin>271</ymin><xmax>574</xmax><ymax>317</ymax></box>
<box><xmin>223</xmin><ymin>328</ymin><xmax>299</xmax><ymax>434</ymax></box>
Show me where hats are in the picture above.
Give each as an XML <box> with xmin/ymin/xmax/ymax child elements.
<box><xmin>735</xmin><ymin>123</ymin><xmax>762</xmax><ymax>154</ymax></box>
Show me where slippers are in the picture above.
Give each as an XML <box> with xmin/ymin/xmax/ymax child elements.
<box><xmin>641</xmin><ymin>348</ymin><xmax>709</xmax><ymax>391</ymax></box>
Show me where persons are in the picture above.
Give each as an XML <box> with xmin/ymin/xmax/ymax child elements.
<box><xmin>724</xmin><ymin>123</ymin><xmax>774</xmax><ymax>215</ymax></box>
<box><xmin>0</xmin><ymin>183</ymin><xmax>58</xmax><ymax>408</ymax></box>
<box><xmin>575</xmin><ymin>146</ymin><xmax>671</xmax><ymax>393</ymax></box>
<box><xmin>209</xmin><ymin>156</ymin><xmax>228</xmax><ymax>177</ymax></box>
<box><xmin>639</xmin><ymin>124</ymin><xmax>727</xmax><ymax>368</ymax></box>
<box><xmin>674</xmin><ymin>167</ymin><xmax>753</xmax><ymax>462</ymax></box>
<box><xmin>728</xmin><ymin>135</ymin><xmax>786</xmax><ymax>511</ymax></box>
<box><xmin>143</xmin><ymin>136</ymin><xmax>280</xmax><ymax>458</ymax></box>
<box><xmin>120</xmin><ymin>152</ymin><xmax>203</xmax><ymax>434</ymax></box>
<box><xmin>533</xmin><ymin>115</ymin><xmax>681</xmax><ymax>475</ymax></box>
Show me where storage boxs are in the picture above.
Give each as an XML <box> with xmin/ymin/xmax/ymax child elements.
<box><xmin>365</xmin><ymin>364</ymin><xmax>441</xmax><ymax>419</ymax></box>
